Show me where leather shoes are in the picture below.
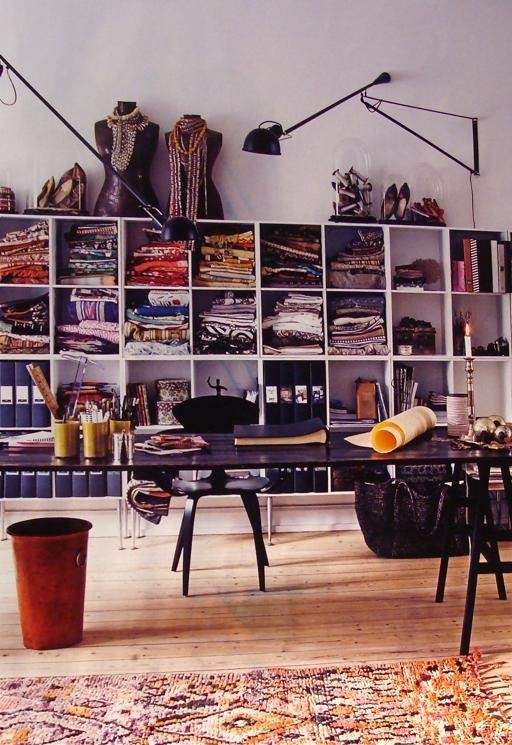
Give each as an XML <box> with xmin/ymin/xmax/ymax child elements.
<box><xmin>381</xmin><ymin>182</ymin><xmax>397</xmax><ymax>220</ymax></box>
<box><xmin>395</xmin><ymin>182</ymin><xmax>410</xmax><ymax>220</ymax></box>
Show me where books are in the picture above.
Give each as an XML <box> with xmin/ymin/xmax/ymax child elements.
<box><xmin>232</xmin><ymin>417</ymin><xmax>330</xmax><ymax>447</ymax></box>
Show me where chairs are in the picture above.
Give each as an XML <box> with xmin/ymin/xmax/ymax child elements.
<box><xmin>169</xmin><ymin>394</ymin><xmax>273</xmax><ymax>596</ymax></box>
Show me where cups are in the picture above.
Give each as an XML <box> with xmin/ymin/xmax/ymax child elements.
<box><xmin>54</xmin><ymin>419</ymin><xmax>135</xmax><ymax>460</ymax></box>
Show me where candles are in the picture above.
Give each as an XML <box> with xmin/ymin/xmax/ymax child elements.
<box><xmin>463</xmin><ymin>324</ymin><xmax>474</xmax><ymax>361</ymax></box>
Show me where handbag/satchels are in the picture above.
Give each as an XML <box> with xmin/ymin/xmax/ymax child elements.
<box><xmin>354</xmin><ymin>475</ymin><xmax>469</xmax><ymax>560</ymax></box>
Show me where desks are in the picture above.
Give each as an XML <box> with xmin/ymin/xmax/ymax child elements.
<box><xmin>0</xmin><ymin>430</ymin><xmax>512</xmax><ymax>652</ymax></box>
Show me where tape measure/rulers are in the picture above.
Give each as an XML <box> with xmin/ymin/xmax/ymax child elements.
<box><xmin>26</xmin><ymin>356</ymin><xmax>88</xmax><ymax>420</ymax></box>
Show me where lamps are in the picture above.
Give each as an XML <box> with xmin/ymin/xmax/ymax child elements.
<box><xmin>240</xmin><ymin>70</ymin><xmax>482</xmax><ymax>180</ymax></box>
<box><xmin>1</xmin><ymin>56</ymin><xmax>200</xmax><ymax>243</ymax></box>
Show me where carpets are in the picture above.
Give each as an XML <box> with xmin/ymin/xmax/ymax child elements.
<box><xmin>1</xmin><ymin>650</ymin><xmax>512</xmax><ymax>743</ymax></box>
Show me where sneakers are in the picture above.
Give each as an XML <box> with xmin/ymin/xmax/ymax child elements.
<box><xmin>407</xmin><ymin>197</ymin><xmax>445</xmax><ymax>224</ymax></box>
<box><xmin>38</xmin><ymin>162</ymin><xmax>87</xmax><ymax>209</ymax></box>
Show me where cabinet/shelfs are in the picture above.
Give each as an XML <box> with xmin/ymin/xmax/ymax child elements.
<box><xmin>0</xmin><ymin>215</ymin><xmax>512</xmax><ymax>541</ymax></box>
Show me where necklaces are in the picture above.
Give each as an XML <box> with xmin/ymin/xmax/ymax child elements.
<box><xmin>167</xmin><ymin>118</ymin><xmax>209</xmax><ymax>219</ymax></box>
<box><xmin>106</xmin><ymin>106</ymin><xmax>148</xmax><ymax>171</ymax></box>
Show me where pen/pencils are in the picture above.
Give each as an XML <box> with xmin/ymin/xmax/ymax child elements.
<box><xmin>85</xmin><ymin>393</ymin><xmax>139</xmax><ymax>421</ymax></box>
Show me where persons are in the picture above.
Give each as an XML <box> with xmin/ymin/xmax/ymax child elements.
<box><xmin>164</xmin><ymin>113</ymin><xmax>225</xmax><ymax>219</ymax></box>
<box><xmin>93</xmin><ymin>100</ymin><xmax>162</xmax><ymax>217</ymax></box>
